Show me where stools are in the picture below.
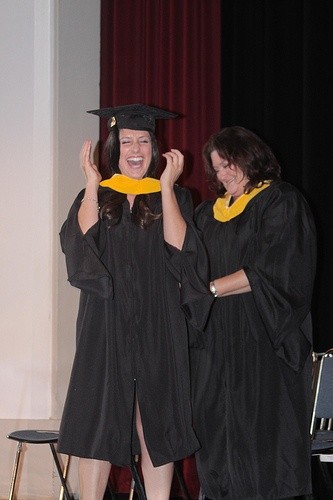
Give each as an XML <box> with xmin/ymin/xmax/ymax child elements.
<box><xmin>6</xmin><ymin>430</ymin><xmax>76</xmax><ymax>500</ymax></box>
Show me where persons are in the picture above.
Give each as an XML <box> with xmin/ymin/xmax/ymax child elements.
<box><xmin>195</xmin><ymin>126</ymin><xmax>318</xmax><ymax>499</ymax></box>
<box><xmin>58</xmin><ymin>101</ymin><xmax>216</xmax><ymax>500</ymax></box>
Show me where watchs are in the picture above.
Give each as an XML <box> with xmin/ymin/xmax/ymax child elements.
<box><xmin>209</xmin><ymin>281</ymin><xmax>218</xmax><ymax>298</ymax></box>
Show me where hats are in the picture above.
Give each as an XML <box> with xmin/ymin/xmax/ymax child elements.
<box><xmin>86</xmin><ymin>104</ymin><xmax>182</xmax><ymax>131</ymax></box>
